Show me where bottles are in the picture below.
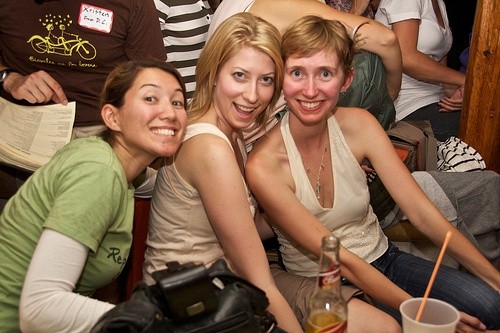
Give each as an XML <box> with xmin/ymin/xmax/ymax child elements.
<box><xmin>305</xmin><ymin>235</ymin><xmax>348</xmax><ymax>333</ymax></box>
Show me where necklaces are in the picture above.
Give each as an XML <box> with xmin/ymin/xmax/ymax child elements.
<box><xmin>302</xmin><ymin>126</ymin><xmax>327</xmax><ymax>200</ymax></box>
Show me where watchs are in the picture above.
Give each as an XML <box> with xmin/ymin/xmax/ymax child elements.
<box><xmin>0</xmin><ymin>69</ymin><xmax>25</xmax><ymax>92</ymax></box>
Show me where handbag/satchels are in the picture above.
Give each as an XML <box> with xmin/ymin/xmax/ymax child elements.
<box><xmin>92</xmin><ymin>259</ymin><xmax>287</xmax><ymax>333</ymax></box>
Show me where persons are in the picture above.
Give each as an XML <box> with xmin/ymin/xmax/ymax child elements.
<box><xmin>140</xmin><ymin>11</ymin><xmax>400</xmax><ymax>333</ymax></box>
<box><xmin>0</xmin><ymin>0</ymin><xmax>500</xmax><ymax>273</ymax></box>
<box><xmin>244</xmin><ymin>15</ymin><xmax>500</xmax><ymax>333</ymax></box>
<box><xmin>0</xmin><ymin>59</ymin><xmax>186</xmax><ymax>333</ymax></box>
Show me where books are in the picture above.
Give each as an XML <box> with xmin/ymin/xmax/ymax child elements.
<box><xmin>0</xmin><ymin>97</ymin><xmax>76</xmax><ymax>174</ymax></box>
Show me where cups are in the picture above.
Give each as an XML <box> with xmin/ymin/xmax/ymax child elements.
<box><xmin>400</xmin><ymin>298</ymin><xmax>460</xmax><ymax>333</ymax></box>
